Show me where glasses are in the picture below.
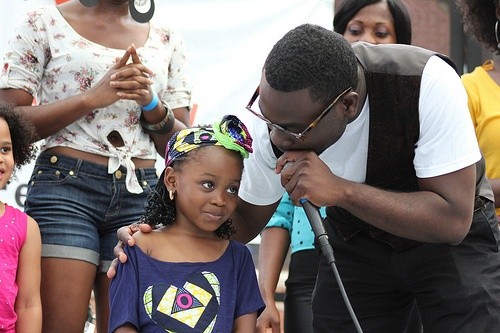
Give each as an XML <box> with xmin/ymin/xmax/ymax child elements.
<box><xmin>246</xmin><ymin>83</ymin><xmax>351</xmax><ymax>141</ymax></box>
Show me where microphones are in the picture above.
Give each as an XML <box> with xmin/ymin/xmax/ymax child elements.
<box><xmin>280</xmin><ymin>162</ymin><xmax>336</xmax><ymax>265</ymax></box>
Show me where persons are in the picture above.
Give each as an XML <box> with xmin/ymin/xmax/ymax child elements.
<box><xmin>456</xmin><ymin>0</ymin><xmax>500</xmax><ymax>229</ymax></box>
<box><xmin>107</xmin><ymin>23</ymin><xmax>500</xmax><ymax>333</ymax></box>
<box><xmin>1</xmin><ymin>103</ymin><xmax>43</xmax><ymax>333</ymax></box>
<box><xmin>256</xmin><ymin>0</ymin><xmax>412</xmax><ymax>333</ymax></box>
<box><xmin>108</xmin><ymin>114</ymin><xmax>267</xmax><ymax>333</ymax></box>
<box><xmin>0</xmin><ymin>0</ymin><xmax>192</xmax><ymax>333</ymax></box>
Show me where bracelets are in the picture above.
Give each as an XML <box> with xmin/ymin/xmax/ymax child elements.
<box><xmin>140</xmin><ymin>92</ymin><xmax>175</xmax><ymax>135</ymax></box>
